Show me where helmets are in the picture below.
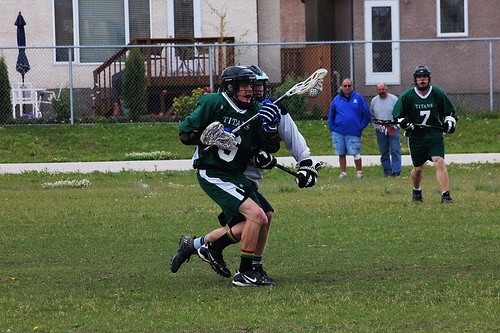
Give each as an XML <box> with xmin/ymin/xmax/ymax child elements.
<box><xmin>245</xmin><ymin>64</ymin><xmax>270</xmax><ymax>101</ymax></box>
<box><xmin>413</xmin><ymin>65</ymin><xmax>431</xmax><ymax>91</ymax></box>
<box><xmin>221</xmin><ymin>65</ymin><xmax>256</xmax><ymax>108</ymax></box>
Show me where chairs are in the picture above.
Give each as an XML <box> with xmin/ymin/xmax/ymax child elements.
<box><xmin>10</xmin><ymin>81</ymin><xmax>64</xmax><ymax>119</ymax></box>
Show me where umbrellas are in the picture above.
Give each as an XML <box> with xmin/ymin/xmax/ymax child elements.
<box><xmin>14</xmin><ymin>12</ymin><xmax>31</xmax><ymax>114</ymax></box>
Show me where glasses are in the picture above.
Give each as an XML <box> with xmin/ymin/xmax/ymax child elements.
<box><xmin>342</xmin><ymin>84</ymin><xmax>352</xmax><ymax>87</ymax></box>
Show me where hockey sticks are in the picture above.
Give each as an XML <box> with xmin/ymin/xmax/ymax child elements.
<box><xmin>203</xmin><ymin>68</ymin><xmax>329</xmax><ymax>153</ymax></box>
<box><xmin>200</xmin><ymin>121</ymin><xmax>299</xmax><ymax>178</ymax></box>
<box><xmin>372</xmin><ymin>118</ymin><xmax>443</xmax><ymax>135</ymax></box>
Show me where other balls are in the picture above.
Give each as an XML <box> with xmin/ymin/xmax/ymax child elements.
<box><xmin>309</xmin><ymin>89</ymin><xmax>319</xmax><ymax>97</ymax></box>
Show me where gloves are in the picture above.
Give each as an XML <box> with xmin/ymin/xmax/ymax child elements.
<box><xmin>400</xmin><ymin>119</ymin><xmax>415</xmax><ymax>132</ymax></box>
<box><xmin>252</xmin><ymin>152</ymin><xmax>277</xmax><ymax>169</ymax></box>
<box><xmin>258</xmin><ymin>102</ymin><xmax>281</xmax><ymax>132</ymax></box>
<box><xmin>295</xmin><ymin>159</ymin><xmax>319</xmax><ymax>188</ymax></box>
<box><xmin>442</xmin><ymin>116</ymin><xmax>457</xmax><ymax>133</ymax></box>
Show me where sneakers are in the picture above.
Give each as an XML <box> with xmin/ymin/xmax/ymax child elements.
<box><xmin>412</xmin><ymin>188</ymin><xmax>423</xmax><ymax>202</ymax></box>
<box><xmin>196</xmin><ymin>242</ymin><xmax>230</xmax><ymax>279</ymax></box>
<box><xmin>356</xmin><ymin>170</ymin><xmax>363</xmax><ymax>178</ymax></box>
<box><xmin>441</xmin><ymin>190</ymin><xmax>453</xmax><ymax>203</ymax></box>
<box><xmin>169</xmin><ymin>235</ymin><xmax>195</xmax><ymax>273</ymax></box>
<box><xmin>253</xmin><ymin>263</ymin><xmax>276</xmax><ymax>286</ymax></box>
<box><xmin>231</xmin><ymin>269</ymin><xmax>265</xmax><ymax>287</ymax></box>
<box><xmin>339</xmin><ymin>173</ymin><xmax>346</xmax><ymax>179</ymax></box>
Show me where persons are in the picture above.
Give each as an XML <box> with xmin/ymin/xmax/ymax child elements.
<box><xmin>179</xmin><ymin>66</ymin><xmax>281</xmax><ymax>288</ymax></box>
<box><xmin>328</xmin><ymin>78</ymin><xmax>371</xmax><ymax>179</ymax></box>
<box><xmin>170</xmin><ymin>65</ymin><xmax>318</xmax><ymax>284</ymax></box>
<box><xmin>369</xmin><ymin>82</ymin><xmax>402</xmax><ymax>177</ymax></box>
<box><xmin>391</xmin><ymin>66</ymin><xmax>458</xmax><ymax>204</ymax></box>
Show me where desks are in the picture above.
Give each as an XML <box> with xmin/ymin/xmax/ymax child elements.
<box><xmin>11</xmin><ymin>89</ymin><xmax>46</xmax><ymax>118</ymax></box>
